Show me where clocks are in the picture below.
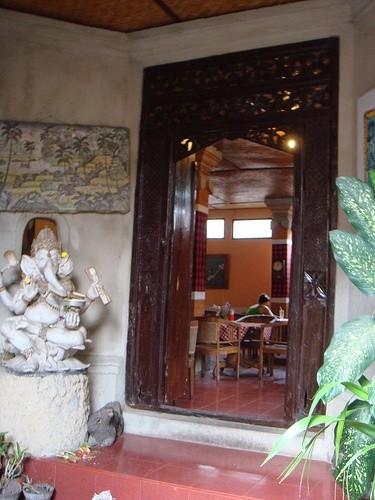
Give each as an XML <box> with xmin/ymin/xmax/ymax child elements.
<box><xmin>273</xmin><ymin>260</ymin><xmax>283</xmax><ymax>271</ymax></box>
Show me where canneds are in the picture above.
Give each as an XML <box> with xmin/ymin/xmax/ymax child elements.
<box><xmin>228</xmin><ymin>309</ymin><xmax>234</xmax><ymax>321</ymax></box>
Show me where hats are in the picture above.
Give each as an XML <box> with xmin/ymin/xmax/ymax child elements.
<box><xmin>259</xmin><ymin>293</ymin><xmax>270</xmax><ymax>305</ymax></box>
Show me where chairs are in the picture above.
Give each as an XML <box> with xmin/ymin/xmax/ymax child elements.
<box><xmin>187</xmin><ymin>320</ymin><xmax>199</xmax><ymax>399</ymax></box>
<box><xmin>192</xmin><ymin>316</ymin><xmax>241</xmax><ymax>384</ymax></box>
<box><xmin>234</xmin><ymin>314</ymin><xmax>277</xmax><ymax>374</ymax></box>
<box><xmin>259</xmin><ymin>323</ymin><xmax>289</xmax><ymax>380</ymax></box>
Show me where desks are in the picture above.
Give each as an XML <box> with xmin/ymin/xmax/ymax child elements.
<box><xmin>220</xmin><ymin>321</ymin><xmax>272</xmax><ymax>346</ymax></box>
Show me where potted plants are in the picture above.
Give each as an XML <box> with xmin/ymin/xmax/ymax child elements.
<box><xmin>0</xmin><ymin>432</ymin><xmax>54</xmax><ymax>500</ymax></box>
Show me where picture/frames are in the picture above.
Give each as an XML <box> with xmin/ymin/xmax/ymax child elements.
<box><xmin>204</xmin><ymin>254</ymin><xmax>229</xmax><ymax>289</ymax></box>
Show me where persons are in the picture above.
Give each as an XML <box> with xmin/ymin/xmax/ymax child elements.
<box><xmin>0</xmin><ymin>226</ymin><xmax>104</xmax><ymax>375</ymax></box>
<box><xmin>245</xmin><ymin>293</ymin><xmax>274</xmax><ymax>317</ymax></box>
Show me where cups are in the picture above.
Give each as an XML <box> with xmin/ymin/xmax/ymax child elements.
<box><xmin>279</xmin><ymin>310</ymin><xmax>284</xmax><ymax>319</ymax></box>
<box><xmin>228</xmin><ymin>315</ymin><xmax>235</xmax><ymax>321</ymax></box>
